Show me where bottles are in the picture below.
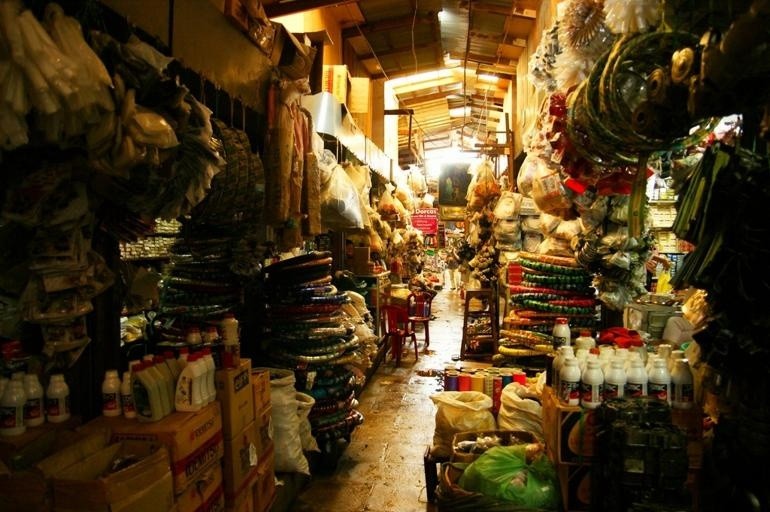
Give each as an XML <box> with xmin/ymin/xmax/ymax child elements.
<box><xmin>550</xmin><ymin>315</ymin><xmax>695</xmax><ymax>412</ymax></box>
<box><xmin>0</xmin><ymin>309</ymin><xmax>241</xmax><ymax>438</ymax></box>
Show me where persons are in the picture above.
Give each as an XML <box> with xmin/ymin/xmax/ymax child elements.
<box><xmin>442</xmin><ymin>229</ymin><xmax>461</xmax><ymax>291</ymax></box>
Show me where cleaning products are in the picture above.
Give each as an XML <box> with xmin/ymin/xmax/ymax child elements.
<box><xmin>0</xmin><ymin>369</ymin><xmax>73</xmax><ymax>437</ymax></box>
<box><xmin>554</xmin><ymin>317</ymin><xmax>695</xmax><ymax>413</ymax></box>
<box><xmin>102</xmin><ymin>367</ymin><xmax>135</xmax><ymax>419</ymax></box>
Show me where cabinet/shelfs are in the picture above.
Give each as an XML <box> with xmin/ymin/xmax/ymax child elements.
<box><xmin>460</xmin><ymin>288</ymin><xmax>498</xmax><ymax>361</ymax></box>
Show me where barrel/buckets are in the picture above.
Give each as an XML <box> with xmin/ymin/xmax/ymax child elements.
<box><xmin>128</xmin><ymin>345</ymin><xmax>214</xmax><ymax>423</ymax></box>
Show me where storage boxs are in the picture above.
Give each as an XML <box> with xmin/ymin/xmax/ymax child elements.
<box><xmin>37</xmin><ymin>357</ymin><xmax>276</xmax><ymax>512</ymax></box>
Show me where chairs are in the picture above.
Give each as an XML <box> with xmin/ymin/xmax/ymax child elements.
<box><xmin>404</xmin><ymin>290</ymin><xmax>437</xmax><ymax>347</ymax></box>
<box><xmin>379</xmin><ymin>304</ymin><xmax>418</xmax><ymax>367</ymax></box>
